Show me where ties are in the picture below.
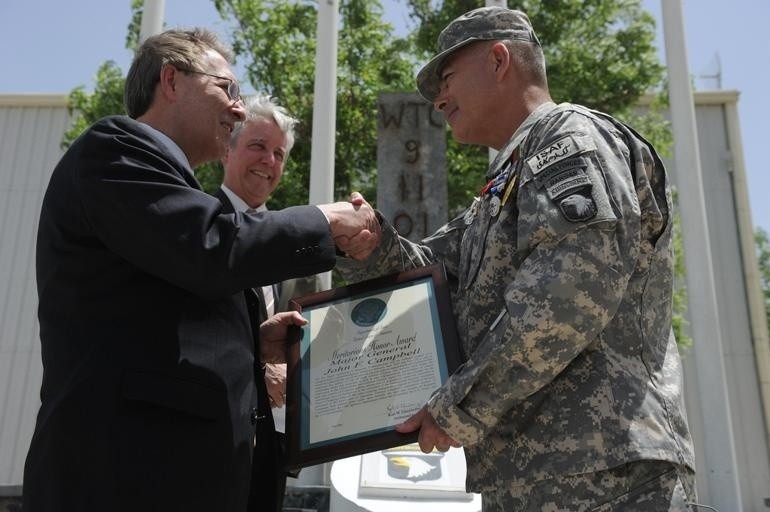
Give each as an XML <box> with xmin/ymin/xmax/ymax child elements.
<box><xmin>262</xmin><ymin>286</ymin><xmax>275</xmax><ymax>319</ymax></box>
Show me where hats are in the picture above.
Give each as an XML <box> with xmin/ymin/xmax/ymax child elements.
<box><xmin>416</xmin><ymin>6</ymin><xmax>542</xmax><ymax>105</ymax></box>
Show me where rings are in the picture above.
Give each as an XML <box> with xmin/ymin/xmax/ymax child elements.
<box><xmin>282</xmin><ymin>393</ymin><xmax>286</xmax><ymax>398</ymax></box>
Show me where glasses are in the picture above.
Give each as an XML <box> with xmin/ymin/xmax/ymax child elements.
<box><xmin>177</xmin><ymin>65</ymin><xmax>249</xmax><ymax>106</ymax></box>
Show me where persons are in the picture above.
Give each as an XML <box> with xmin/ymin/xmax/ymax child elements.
<box><xmin>332</xmin><ymin>3</ymin><xmax>701</xmax><ymax>509</ymax></box>
<box><xmin>14</xmin><ymin>20</ymin><xmax>382</xmax><ymax>509</ymax></box>
<box><xmin>194</xmin><ymin>89</ymin><xmax>300</xmax><ymax>509</ymax></box>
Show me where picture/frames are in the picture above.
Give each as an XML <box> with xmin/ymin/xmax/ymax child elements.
<box><xmin>284</xmin><ymin>262</ymin><xmax>465</xmax><ymax>471</ymax></box>
